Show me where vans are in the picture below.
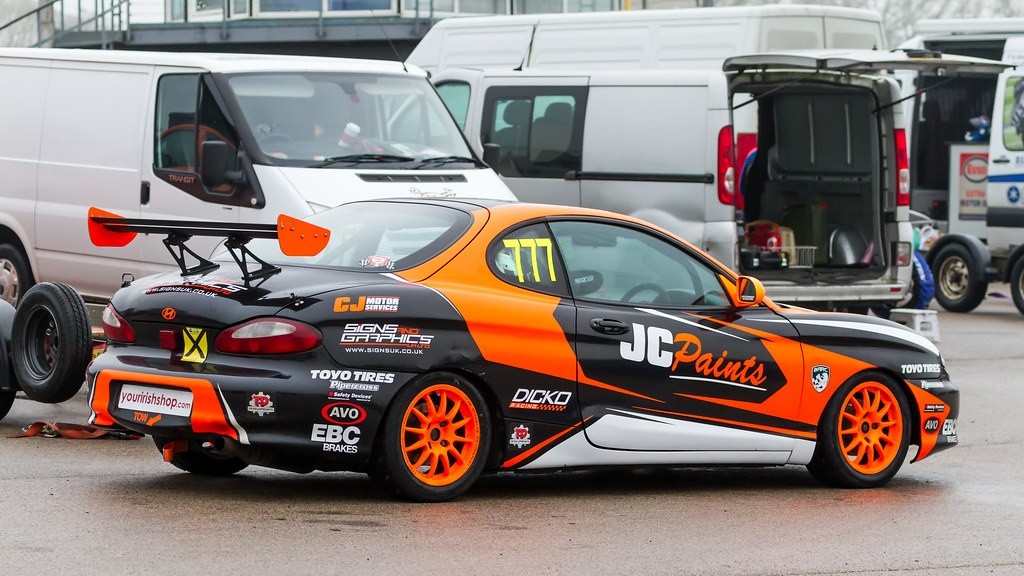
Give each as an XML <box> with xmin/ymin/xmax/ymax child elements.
<box><xmin>340</xmin><ymin>2</ymin><xmax>1019</xmax><ymax>314</ymax></box>
<box><xmin>979</xmin><ymin>31</ymin><xmax>1024</xmax><ymax>320</ymax></box>
<box><xmin>887</xmin><ymin>15</ymin><xmax>1024</xmax><ymax>315</ymax></box>
<box><xmin>0</xmin><ymin>42</ymin><xmax>542</xmax><ymax>432</ymax></box>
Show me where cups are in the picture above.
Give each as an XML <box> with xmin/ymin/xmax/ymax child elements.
<box><xmin>338</xmin><ymin>122</ymin><xmax>360</xmax><ymax>148</ymax></box>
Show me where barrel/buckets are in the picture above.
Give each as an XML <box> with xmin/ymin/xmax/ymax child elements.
<box><xmin>788</xmin><ymin>198</ymin><xmax>829</xmax><ymax>266</ymax></box>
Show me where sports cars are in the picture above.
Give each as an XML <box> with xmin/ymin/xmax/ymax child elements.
<box><xmin>84</xmin><ymin>199</ymin><xmax>967</xmax><ymax>506</ymax></box>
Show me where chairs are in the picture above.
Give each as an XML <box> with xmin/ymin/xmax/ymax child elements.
<box><xmin>531</xmin><ymin>101</ymin><xmax>574</xmax><ymax>164</ymax></box>
<box><xmin>494</xmin><ymin>102</ymin><xmax>531</xmax><ymax>160</ymax></box>
